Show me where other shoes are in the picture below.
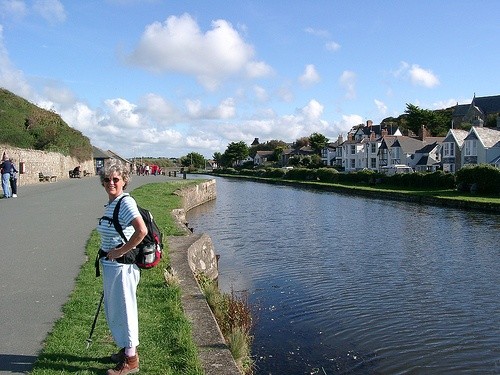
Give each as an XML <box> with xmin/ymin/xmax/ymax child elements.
<box><xmin>12</xmin><ymin>194</ymin><xmax>17</xmax><ymax>197</ymax></box>
<box><xmin>0</xmin><ymin>196</ymin><xmax>10</xmax><ymax>199</ymax></box>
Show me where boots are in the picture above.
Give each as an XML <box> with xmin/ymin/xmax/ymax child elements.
<box><xmin>107</xmin><ymin>355</ymin><xmax>140</xmax><ymax>374</ymax></box>
<box><xmin>111</xmin><ymin>349</ymin><xmax>140</xmax><ymax>364</ymax></box>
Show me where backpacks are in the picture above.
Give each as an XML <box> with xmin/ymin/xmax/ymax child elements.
<box><xmin>98</xmin><ymin>195</ymin><xmax>163</xmax><ymax>269</ymax></box>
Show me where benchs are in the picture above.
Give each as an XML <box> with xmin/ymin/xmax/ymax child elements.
<box><xmin>69</xmin><ymin>170</ymin><xmax>81</xmax><ymax>178</ymax></box>
<box><xmin>39</xmin><ymin>173</ymin><xmax>57</xmax><ymax>182</ymax></box>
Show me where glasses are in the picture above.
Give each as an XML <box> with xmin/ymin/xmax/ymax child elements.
<box><xmin>104</xmin><ymin>177</ymin><xmax>122</xmax><ymax>184</ymax></box>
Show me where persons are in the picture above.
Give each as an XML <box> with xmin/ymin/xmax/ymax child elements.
<box><xmin>138</xmin><ymin>163</ymin><xmax>161</xmax><ymax>176</ymax></box>
<box><xmin>94</xmin><ymin>158</ymin><xmax>148</xmax><ymax>374</ymax></box>
<box><xmin>0</xmin><ymin>154</ymin><xmax>18</xmax><ymax>199</ymax></box>
<box><xmin>73</xmin><ymin>166</ymin><xmax>81</xmax><ymax>178</ymax></box>
